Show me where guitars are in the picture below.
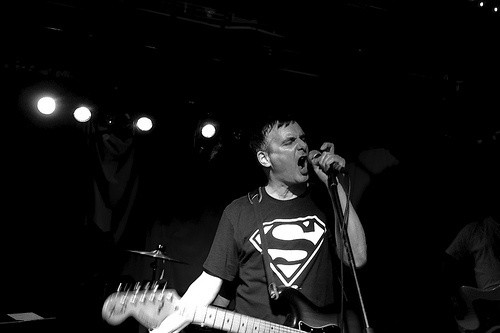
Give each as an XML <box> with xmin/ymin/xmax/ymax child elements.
<box><xmin>103</xmin><ymin>281</ymin><xmax>341</xmax><ymax>333</ymax></box>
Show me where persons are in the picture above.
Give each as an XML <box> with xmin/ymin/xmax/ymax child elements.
<box><xmin>148</xmin><ymin>107</ymin><xmax>368</xmax><ymax>333</ymax></box>
<box><xmin>443</xmin><ymin>196</ymin><xmax>500</xmax><ymax>333</ymax></box>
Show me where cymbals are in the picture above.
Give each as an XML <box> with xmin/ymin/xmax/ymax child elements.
<box><xmin>128</xmin><ymin>250</ymin><xmax>190</xmax><ymax>265</ymax></box>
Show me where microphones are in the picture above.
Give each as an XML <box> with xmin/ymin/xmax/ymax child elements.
<box><xmin>307</xmin><ymin>150</ymin><xmax>349</xmax><ymax>176</ymax></box>
